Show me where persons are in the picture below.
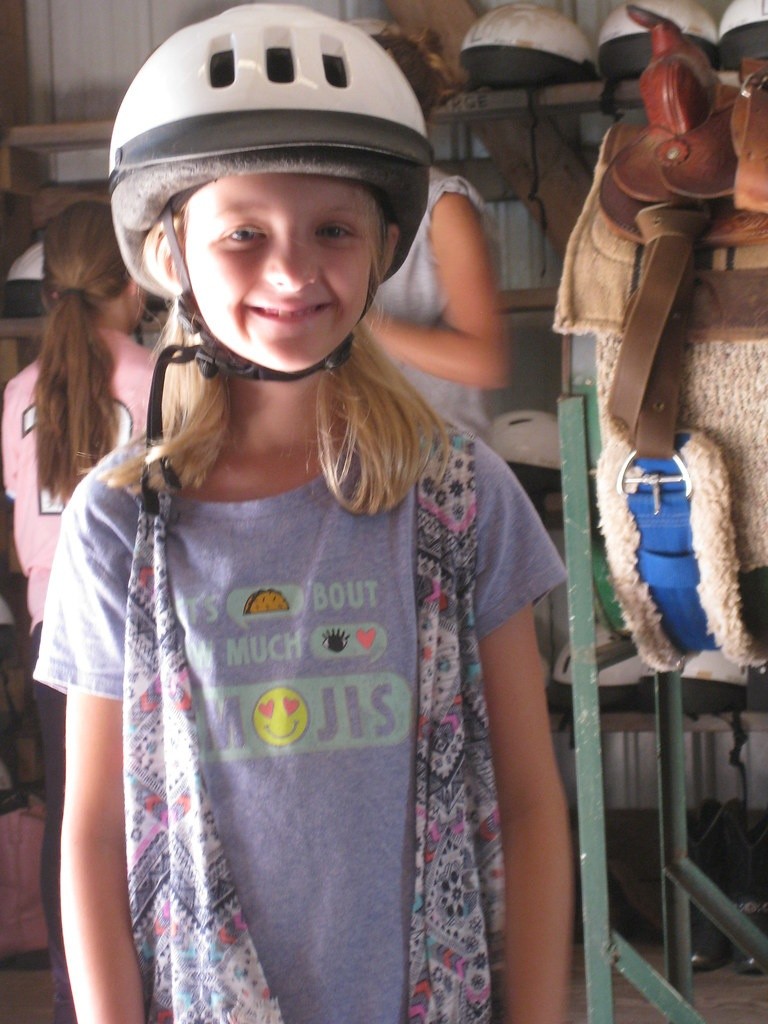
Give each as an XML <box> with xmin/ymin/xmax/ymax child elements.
<box><xmin>347</xmin><ymin>30</ymin><xmax>511</xmax><ymax>444</ymax></box>
<box><xmin>22</xmin><ymin>3</ymin><xmax>573</xmax><ymax>1024</ymax></box>
<box><xmin>0</xmin><ymin>194</ymin><xmax>156</xmax><ymax>1024</ymax></box>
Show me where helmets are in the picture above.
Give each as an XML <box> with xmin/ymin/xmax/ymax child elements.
<box><xmin>484</xmin><ymin>408</ymin><xmax>563</xmax><ymax>481</ymax></box>
<box><xmin>597</xmin><ymin>0</ymin><xmax>720</xmax><ymax>76</ymax></box>
<box><xmin>460</xmin><ymin>2</ymin><xmax>597</xmax><ymax>91</ymax></box>
<box><xmin>544</xmin><ymin>621</ymin><xmax>750</xmax><ymax>714</ymax></box>
<box><xmin>0</xmin><ymin>241</ymin><xmax>45</xmax><ymax>317</ymax></box>
<box><xmin>720</xmin><ymin>0</ymin><xmax>768</xmax><ymax>66</ymax></box>
<box><xmin>108</xmin><ymin>4</ymin><xmax>432</xmax><ymax>304</ymax></box>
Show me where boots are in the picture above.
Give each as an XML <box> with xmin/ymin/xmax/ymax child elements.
<box><xmin>724</xmin><ymin>811</ymin><xmax>768</xmax><ymax>974</ymax></box>
<box><xmin>691</xmin><ymin>800</ymin><xmax>737</xmax><ymax>968</ymax></box>
